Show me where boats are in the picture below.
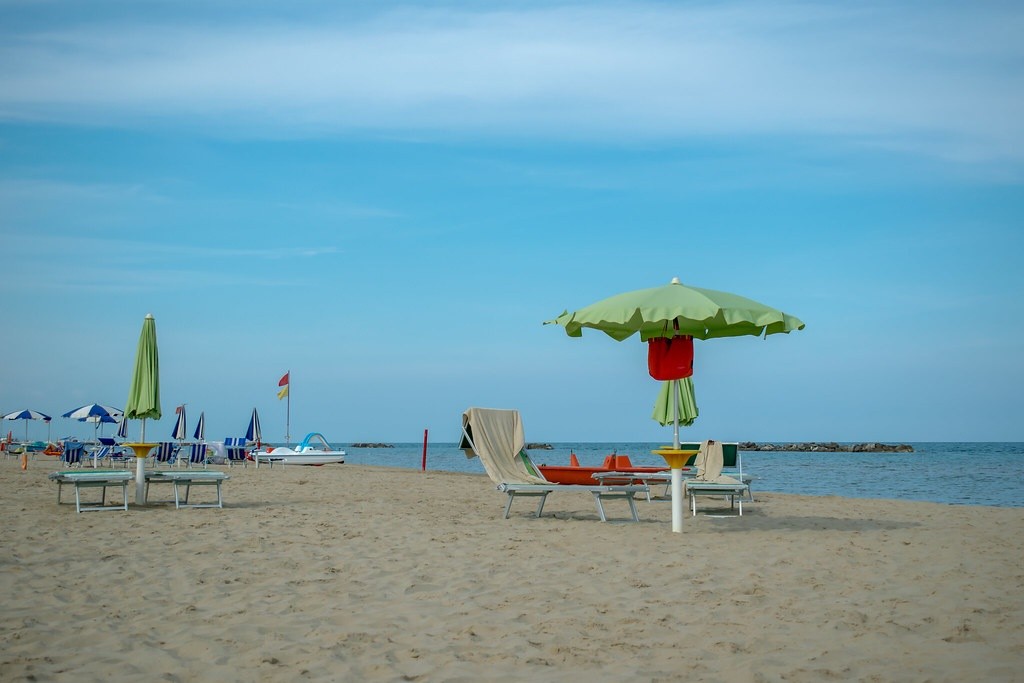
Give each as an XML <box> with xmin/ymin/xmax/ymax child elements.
<box><xmin>533</xmin><ymin>448</ymin><xmax>692</xmax><ymax>486</ymax></box>
<box><xmin>245</xmin><ymin>432</ymin><xmax>347</xmax><ymax>465</ymax></box>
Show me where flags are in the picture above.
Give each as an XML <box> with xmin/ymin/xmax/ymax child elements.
<box><xmin>279</xmin><ymin>374</ymin><xmax>288</xmax><ymax>386</ymax></box>
<box><xmin>278</xmin><ymin>385</ymin><xmax>288</xmax><ymax>400</ymax></box>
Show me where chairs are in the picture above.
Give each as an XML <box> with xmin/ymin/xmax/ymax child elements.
<box><xmin>4</xmin><ymin>438</ymin><xmax>288</xmax><ymax>512</ymax></box>
<box><xmin>460</xmin><ymin>408</ymin><xmax>762</xmax><ymax>524</ymax></box>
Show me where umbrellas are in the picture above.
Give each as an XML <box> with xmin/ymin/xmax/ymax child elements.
<box><xmin>60</xmin><ymin>402</ymin><xmax>124</xmax><ymax>448</ymax></box>
<box><xmin>172</xmin><ymin>407</ymin><xmax>186</xmax><ymax>445</ymax></box>
<box><xmin>124</xmin><ymin>314</ymin><xmax>162</xmax><ymax>444</ymax></box>
<box><xmin>194</xmin><ymin>413</ymin><xmax>205</xmax><ymax>440</ymax></box>
<box><xmin>245</xmin><ymin>408</ymin><xmax>262</xmax><ymax>441</ymax></box>
<box><xmin>651</xmin><ymin>378</ymin><xmax>699</xmax><ymax>428</ymax></box>
<box><xmin>543</xmin><ymin>277</ymin><xmax>805</xmax><ymax>442</ymax></box>
<box><xmin>117</xmin><ymin>418</ymin><xmax>128</xmax><ymax>442</ymax></box>
<box><xmin>0</xmin><ymin>409</ymin><xmax>52</xmax><ymax>441</ymax></box>
